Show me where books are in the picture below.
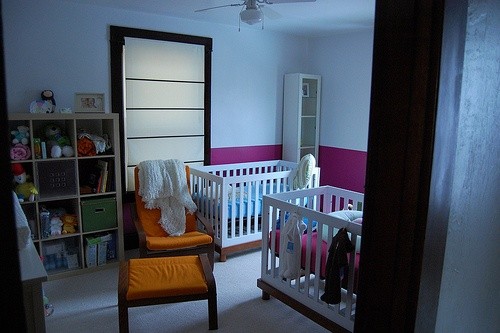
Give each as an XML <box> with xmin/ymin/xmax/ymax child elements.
<box><xmin>80</xmin><ymin>159</ymin><xmax>116</xmax><ymax>194</ymax></box>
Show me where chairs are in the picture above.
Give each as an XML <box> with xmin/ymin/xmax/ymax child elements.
<box><xmin>134</xmin><ymin>160</ymin><xmax>215</xmax><ymax>271</ymax></box>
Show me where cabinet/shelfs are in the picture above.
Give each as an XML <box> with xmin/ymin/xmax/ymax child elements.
<box><xmin>282</xmin><ymin>73</ymin><xmax>322</xmax><ymax>167</ymax></box>
<box><xmin>7</xmin><ymin>112</ymin><xmax>124</xmax><ymax>281</ymax></box>
<box><xmin>20</xmin><ymin>238</ymin><xmax>48</xmax><ymax>333</ymax></box>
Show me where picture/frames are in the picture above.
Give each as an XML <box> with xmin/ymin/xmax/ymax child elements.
<box><xmin>302</xmin><ymin>83</ymin><xmax>309</xmax><ymax>97</ymax></box>
<box><xmin>75</xmin><ymin>93</ymin><xmax>105</xmax><ymax>113</ymax></box>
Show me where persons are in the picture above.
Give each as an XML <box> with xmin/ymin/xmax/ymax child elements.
<box><xmin>81</xmin><ymin>98</ymin><xmax>97</xmax><ymax>108</ymax></box>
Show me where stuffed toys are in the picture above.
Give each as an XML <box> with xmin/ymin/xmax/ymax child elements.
<box><xmin>45</xmin><ymin>122</ymin><xmax>74</xmax><ymax>158</ymax></box>
<box><xmin>11</xmin><ymin>163</ymin><xmax>39</xmax><ymax>203</ymax></box>
<box><xmin>50</xmin><ymin>216</ymin><xmax>64</xmax><ymax>235</ymax></box>
<box><xmin>62</xmin><ymin>212</ymin><xmax>77</xmax><ymax>234</ymax></box>
<box><xmin>11</xmin><ymin>126</ymin><xmax>29</xmax><ymax>145</ymax></box>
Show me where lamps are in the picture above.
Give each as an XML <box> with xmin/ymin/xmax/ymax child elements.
<box><xmin>239</xmin><ymin>10</ymin><xmax>264</xmax><ymax>31</ymax></box>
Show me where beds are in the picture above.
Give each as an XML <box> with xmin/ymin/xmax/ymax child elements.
<box><xmin>189</xmin><ymin>160</ymin><xmax>321</xmax><ymax>262</ymax></box>
<box><xmin>258</xmin><ymin>185</ymin><xmax>364</xmax><ymax>333</ymax></box>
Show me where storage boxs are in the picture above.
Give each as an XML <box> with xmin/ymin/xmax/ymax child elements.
<box><xmin>80</xmin><ymin>197</ymin><xmax>117</xmax><ymax>231</ymax></box>
<box><xmin>84</xmin><ymin>233</ymin><xmax>116</xmax><ymax>267</ymax></box>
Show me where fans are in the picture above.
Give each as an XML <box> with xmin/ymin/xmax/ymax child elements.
<box><xmin>194</xmin><ymin>0</ymin><xmax>316</xmax><ymax>19</ymax></box>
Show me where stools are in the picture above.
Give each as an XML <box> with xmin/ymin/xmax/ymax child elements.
<box><xmin>119</xmin><ymin>253</ymin><xmax>219</xmax><ymax>333</ymax></box>
